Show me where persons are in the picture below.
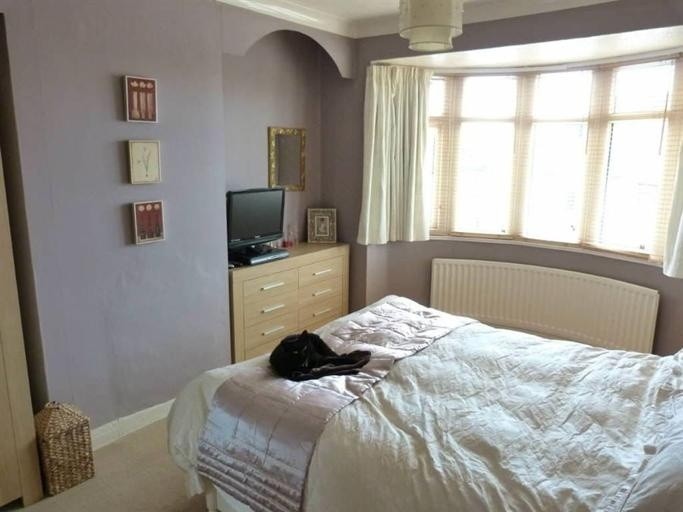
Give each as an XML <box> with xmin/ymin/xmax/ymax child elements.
<box><xmin>318</xmin><ymin>218</ymin><xmax>326</xmax><ymax>233</ymax></box>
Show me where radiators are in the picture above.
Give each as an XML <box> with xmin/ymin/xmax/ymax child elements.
<box><xmin>429</xmin><ymin>257</ymin><xmax>661</xmax><ymax>354</ymax></box>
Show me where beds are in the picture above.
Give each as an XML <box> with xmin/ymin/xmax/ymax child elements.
<box><xmin>164</xmin><ymin>292</ymin><xmax>683</xmax><ymax>512</ymax></box>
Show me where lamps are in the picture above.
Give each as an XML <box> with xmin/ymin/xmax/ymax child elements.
<box><xmin>395</xmin><ymin>1</ymin><xmax>463</xmax><ymax>52</ymax></box>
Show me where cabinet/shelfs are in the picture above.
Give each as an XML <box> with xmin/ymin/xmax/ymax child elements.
<box><xmin>228</xmin><ymin>241</ymin><xmax>350</xmax><ymax>364</ymax></box>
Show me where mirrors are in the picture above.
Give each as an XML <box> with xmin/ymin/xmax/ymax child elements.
<box><xmin>266</xmin><ymin>126</ymin><xmax>306</xmax><ymax>192</ymax></box>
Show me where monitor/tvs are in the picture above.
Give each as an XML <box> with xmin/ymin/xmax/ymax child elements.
<box><xmin>226</xmin><ymin>187</ymin><xmax>290</xmax><ymax>266</ymax></box>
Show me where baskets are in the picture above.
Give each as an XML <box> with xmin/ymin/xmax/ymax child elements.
<box><xmin>36</xmin><ymin>400</ymin><xmax>95</xmax><ymax>494</ymax></box>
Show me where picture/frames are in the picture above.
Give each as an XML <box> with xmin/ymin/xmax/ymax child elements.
<box><xmin>122</xmin><ymin>74</ymin><xmax>165</xmax><ymax>247</ymax></box>
<box><xmin>306</xmin><ymin>207</ymin><xmax>337</xmax><ymax>244</ymax></box>
